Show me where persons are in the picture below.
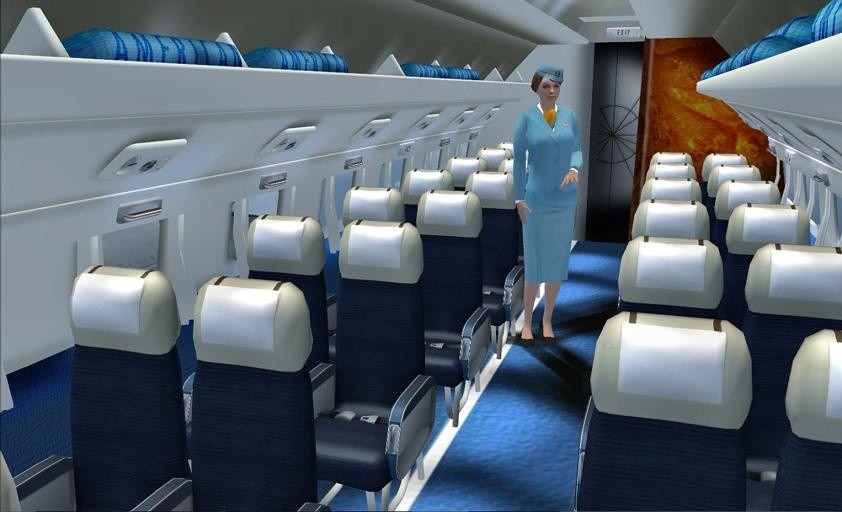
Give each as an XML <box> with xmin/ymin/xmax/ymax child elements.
<box><xmin>512</xmin><ymin>65</ymin><xmax>584</xmax><ymax>348</ymax></box>
